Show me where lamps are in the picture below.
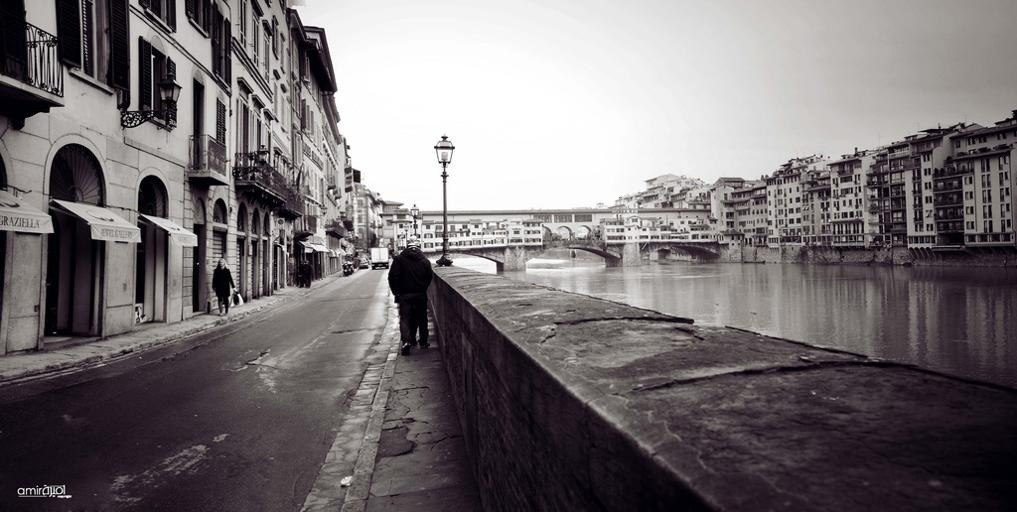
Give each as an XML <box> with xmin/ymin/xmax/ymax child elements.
<box><xmin>121</xmin><ymin>73</ymin><xmax>182</xmax><ymax>130</ymax></box>
<box><xmin>232</xmin><ymin>144</ymin><xmax>269</xmax><ymax>177</ymax></box>
<box><xmin>304</xmin><ymin>204</ymin><xmax>328</xmax><ymax>219</ymax></box>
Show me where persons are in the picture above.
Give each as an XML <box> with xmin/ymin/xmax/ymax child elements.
<box><xmin>211</xmin><ymin>258</ymin><xmax>236</xmax><ymax>315</ymax></box>
<box><xmin>388</xmin><ymin>235</ymin><xmax>434</xmax><ymax>356</ymax></box>
<box><xmin>297</xmin><ymin>262</ymin><xmax>306</xmax><ymax>287</ymax></box>
<box><xmin>303</xmin><ymin>261</ymin><xmax>312</xmax><ymax>288</ymax></box>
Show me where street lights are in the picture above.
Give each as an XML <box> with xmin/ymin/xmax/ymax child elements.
<box><xmin>433</xmin><ymin>133</ymin><xmax>456</xmax><ymax>266</ymax></box>
<box><xmin>410</xmin><ymin>203</ymin><xmax>420</xmax><ymax>242</ymax></box>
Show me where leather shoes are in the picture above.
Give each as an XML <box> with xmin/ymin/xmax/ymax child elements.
<box><xmin>402</xmin><ymin>342</ymin><xmax>430</xmax><ymax>356</ymax></box>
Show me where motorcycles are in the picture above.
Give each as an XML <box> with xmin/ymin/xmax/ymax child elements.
<box><xmin>341</xmin><ymin>261</ymin><xmax>354</xmax><ymax>276</ymax></box>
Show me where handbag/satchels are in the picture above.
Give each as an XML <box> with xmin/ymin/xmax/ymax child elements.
<box><xmin>234</xmin><ymin>293</ymin><xmax>239</xmax><ymax>305</ymax></box>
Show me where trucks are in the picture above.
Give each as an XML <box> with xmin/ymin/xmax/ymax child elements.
<box><xmin>369</xmin><ymin>246</ymin><xmax>390</xmax><ymax>270</ymax></box>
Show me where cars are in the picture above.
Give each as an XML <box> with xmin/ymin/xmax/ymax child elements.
<box><xmin>357</xmin><ymin>257</ymin><xmax>369</xmax><ymax>269</ymax></box>
